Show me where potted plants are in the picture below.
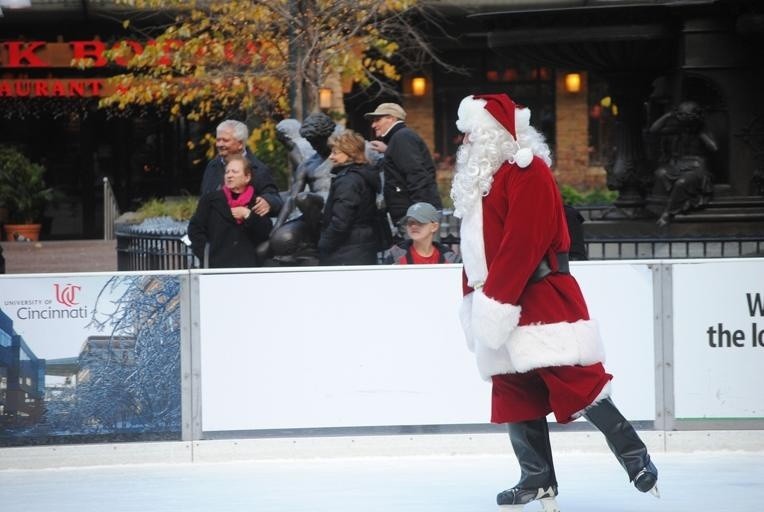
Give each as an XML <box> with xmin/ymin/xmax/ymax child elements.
<box><xmin>0</xmin><ymin>142</ymin><xmax>67</xmax><ymax>241</ymax></box>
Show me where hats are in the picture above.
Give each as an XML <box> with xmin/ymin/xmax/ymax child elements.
<box><xmin>454</xmin><ymin>94</ymin><xmax>533</xmax><ymax>170</ymax></box>
<box><xmin>363</xmin><ymin>103</ymin><xmax>406</xmax><ymax>121</ymax></box>
<box><xmin>399</xmin><ymin>202</ymin><xmax>441</xmax><ymax>225</ymax></box>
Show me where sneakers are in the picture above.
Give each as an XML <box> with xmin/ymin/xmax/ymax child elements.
<box><xmin>497</xmin><ymin>484</ymin><xmax>559</xmax><ymax>505</ymax></box>
<box><xmin>633</xmin><ymin>461</ymin><xmax>659</xmax><ymax>493</ymax></box>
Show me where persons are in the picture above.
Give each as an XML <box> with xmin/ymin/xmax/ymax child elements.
<box><xmin>314</xmin><ymin>129</ymin><xmax>381</xmax><ymax>266</ymax></box>
<box><xmin>647</xmin><ymin>102</ymin><xmax>719</xmax><ymax>227</ymax></box>
<box><xmin>448</xmin><ymin>93</ymin><xmax>660</xmax><ymax>506</ymax></box>
<box><xmin>272</xmin><ymin>118</ymin><xmax>317</xmax><ymax>201</ymax></box>
<box><xmin>395</xmin><ymin>201</ymin><xmax>450</xmax><ymax>265</ymax></box>
<box><xmin>187</xmin><ymin>154</ymin><xmax>273</xmax><ymax>267</ymax></box>
<box><xmin>266</xmin><ymin>112</ymin><xmax>337</xmax><ymax>260</ymax></box>
<box><xmin>198</xmin><ymin>118</ymin><xmax>284</xmax><ymax>217</ymax></box>
<box><xmin>361</xmin><ymin>102</ymin><xmax>444</xmax><ymax>246</ymax></box>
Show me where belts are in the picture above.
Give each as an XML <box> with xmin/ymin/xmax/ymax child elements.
<box><xmin>528</xmin><ymin>252</ymin><xmax>571</xmax><ymax>284</ymax></box>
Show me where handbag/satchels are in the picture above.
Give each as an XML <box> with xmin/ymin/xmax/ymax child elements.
<box><xmin>374</xmin><ymin>205</ymin><xmax>393</xmax><ymax>252</ymax></box>
